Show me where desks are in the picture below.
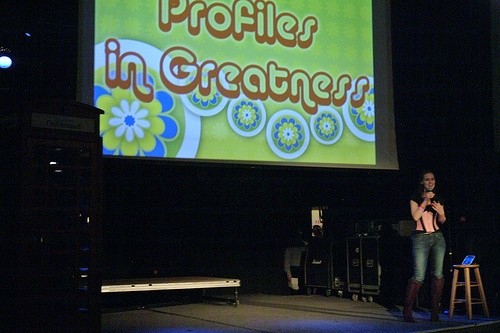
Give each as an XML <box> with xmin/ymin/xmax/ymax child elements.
<box><xmin>100</xmin><ymin>277</ymin><xmax>241</xmax><ymax>307</ymax></box>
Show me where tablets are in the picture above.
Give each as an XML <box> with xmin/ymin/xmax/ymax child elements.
<box><xmin>461</xmin><ymin>255</ymin><xmax>476</xmax><ymax>265</ymax></box>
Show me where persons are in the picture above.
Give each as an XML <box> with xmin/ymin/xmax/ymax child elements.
<box><xmin>401</xmin><ymin>167</ymin><xmax>446</xmax><ymax>323</ymax></box>
<box><xmin>284</xmin><ymin>226</ymin><xmax>323</xmax><ymax>295</ymax></box>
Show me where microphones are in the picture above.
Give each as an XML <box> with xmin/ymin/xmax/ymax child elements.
<box><xmin>428</xmin><ymin>189</ymin><xmax>435</xmax><ymax>203</ymax></box>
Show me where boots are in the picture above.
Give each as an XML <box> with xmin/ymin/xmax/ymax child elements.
<box><xmin>402</xmin><ymin>279</ymin><xmax>422</xmax><ymax>323</ymax></box>
<box><xmin>430</xmin><ymin>277</ymin><xmax>444</xmax><ymax>322</ymax></box>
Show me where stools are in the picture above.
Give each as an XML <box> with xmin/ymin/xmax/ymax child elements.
<box><xmin>449</xmin><ymin>264</ymin><xmax>490</xmax><ymax>320</ymax></box>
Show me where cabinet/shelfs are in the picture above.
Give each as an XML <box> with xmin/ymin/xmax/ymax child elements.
<box><xmin>0</xmin><ymin>101</ymin><xmax>105</xmax><ymax>333</ymax></box>
